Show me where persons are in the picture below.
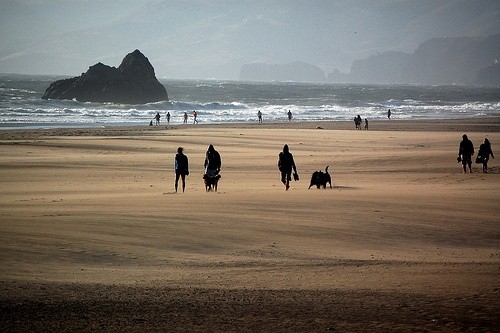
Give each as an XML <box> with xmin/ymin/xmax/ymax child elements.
<box><xmin>192</xmin><ymin>111</ymin><xmax>198</xmax><ymax>124</ymax></box>
<box><xmin>167</xmin><ymin>112</ymin><xmax>170</xmax><ymax>124</ymax></box>
<box><xmin>278</xmin><ymin>144</ymin><xmax>297</xmax><ymax>191</ymax></box>
<box><xmin>204</xmin><ymin>144</ymin><xmax>221</xmax><ymax>192</ymax></box>
<box><xmin>258</xmin><ymin>110</ymin><xmax>262</xmax><ymax>124</ymax></box>
<box><xmin>153</xmin><ymin>113</ymin><xmax>160</xmax><ymax>126</ymax></box>
<box><xmin>184</xmin><ymin>113</ymin><xmax>187</xmax><ymax>124</ymax></box>
<box><xmin>459</xmin><ymin>134</ymin><xmax>474</xmax><ymax>174</ymax></box>
<box><xmin>388</xmin><ymin>109</ymin><xmax>391</xmax><ymax>119</ymax></box>
<box><xmin>354</xmin><ymin>114</ymin><xmax>369</xmax><ymax>130</ymax></box>
<box><xmin>480</xmin><ymin>139</ymin><xmax>494</xmax><ymax>173</ymax></box>
<box><xmin>287</xmin><ymin>110</ymin><xmax>292</xmax><ymax>122</ymax></box>
<box><xmin>175</xmin><ymin>146</ymin><xmax>188</xmax><ymax>193</ymax></box>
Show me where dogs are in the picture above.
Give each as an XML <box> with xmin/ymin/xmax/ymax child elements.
<box><xmin>308</xmin><ymin>166</ymin><xmax>331</xmax><ymax>189</ymax></box>
<box><xmin>203</xmin><ymin>174</ymin><xmax>221</xmax><ymax>192</ymax></box>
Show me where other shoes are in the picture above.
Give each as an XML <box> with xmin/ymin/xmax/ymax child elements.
<box><xmin>293</xmin><ymin>174</ymin><xmax>299</xmax><ymax>181</ymax></box>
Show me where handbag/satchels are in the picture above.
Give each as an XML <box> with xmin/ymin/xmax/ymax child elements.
<box><xmin>476</xmin><ymin>156</ymin><xmax>481</xmax><ymax>164</ymax></box>
<box><xmin>457</xmin><ymin>157</ymin><xmax>462</xmax><ymax>163</ymax></box>
